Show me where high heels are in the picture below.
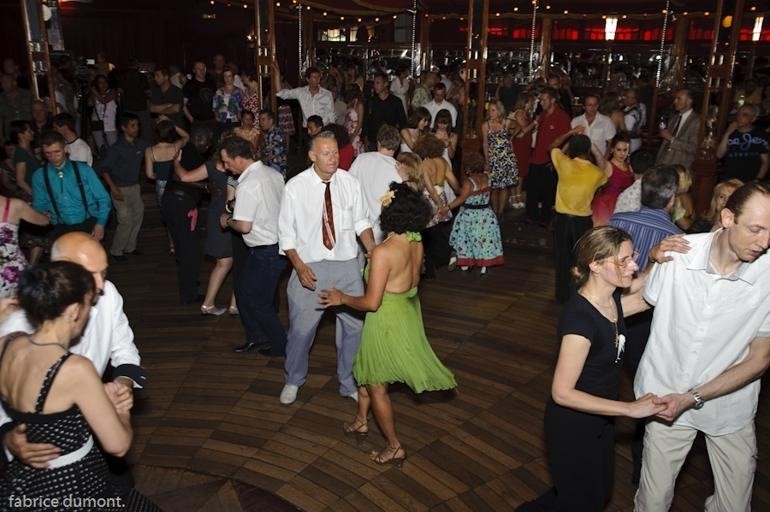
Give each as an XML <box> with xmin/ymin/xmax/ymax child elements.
<box><xmin>343</xmin><ymin>416</ymin><xmax>368</xmax><ymax>442</ymax></box>
<box><xmin>368</xmin><ymin>442</ymin><xmax>406</xmax><ymax>468</ymax></box>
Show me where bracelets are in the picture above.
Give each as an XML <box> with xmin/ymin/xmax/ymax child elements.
<box><xmin>647</xmin><ymin>243</ymin><xmax>659</xmax><ymax>264</ymax></box>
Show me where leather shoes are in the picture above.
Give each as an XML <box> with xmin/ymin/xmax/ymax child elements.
<box><xmin>233</xmin><ymin>341</ymin><xmax>256</xmax><ymax>353</ymax></box>
<box><xmin>508</xmin><ymin>196</ymin><xmax>520</xmax><ymax>209</ymax></box>
<box><xmin>279</xmin><ymin>384</ymin><xmax>299</xmax><ymax>405</ymax></box>
<box><xmin>200</xmin><ymin>305</ymin><xmax>227</xmax><ymax>316</ymax></box>
<box><xmin>516</xmin><ymin>195</ymin><xmax>526</xmax><ymax>209</ymax></box>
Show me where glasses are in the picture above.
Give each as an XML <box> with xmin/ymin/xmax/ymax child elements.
<box><xmin>607</xmin><ymin>249</ymin><xmax>640</xmax><ymax>268</ymax></box>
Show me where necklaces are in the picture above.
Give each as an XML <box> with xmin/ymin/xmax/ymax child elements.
<box><xmin>28</xmin><ymin>334</ymin><xmax>67</xmax><ymax>354</ymax></box>
<box><xmin>576</xmin><ymin>290</ymin><xmax>622</xmax><ymax>350</ymax></box>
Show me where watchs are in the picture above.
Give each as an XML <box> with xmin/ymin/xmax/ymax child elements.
<box><xmin>689</xmin><ymin>388</ymin><xmax>706</xmax><ymax>410</ymax></box>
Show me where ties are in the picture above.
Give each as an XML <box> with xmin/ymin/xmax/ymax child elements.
<box><xmin>665</xmin><ymin>116</ymin><xmax>682</xmax><ymax>151</ymax></box>
<box><xmin>322</xmin><ymin>181</ymin><xmax>336</xmax><ymax>250</ymax></box>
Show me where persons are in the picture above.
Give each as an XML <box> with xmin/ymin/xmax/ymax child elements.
<box><xmin>547</xmin><ymin>125</ymin><xmax>616</xmax><ymax>302</ymax></box>
<box><xmin>621</xmin><ymin>181</ymin><xmax>769</xmax><ymax>510</ymax></box>
<box><xmin>513</xmin><ymin>69</ymin><xmax>769</xmax><ymax>232</ymax></box>
<box><xmin>0</xmin><ymin>229</ymin><xmax>148</xmax><ymax>472</ymax></box>
<box><xmin>0</xmin><ymin>263</ymin><xmax>164</xmax><ymax>511</ymax></box>
<box><xmin>313</xmin><ymin>177</ymin><xmax>459</xmax><ymax>464</ymax></box>
<box><xmin>515</xmin><ymin>225</ymin><xmax>691</xmax><ymax>511</ymax></box>
<box><xmin>610</xmin><ymin>163</ymin><xmax>689</xmax><ymax>486</ymax></box>
<box><xmin>1</xmin><ymin>54</ymin><xmax>514</xmax><ymax>404</ymax></box>
